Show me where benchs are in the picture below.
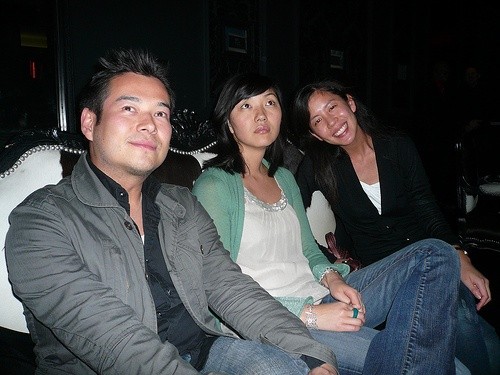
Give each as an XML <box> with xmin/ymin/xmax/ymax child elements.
<box><xmin>1</xmin><ymin>126</ymin><xmax>499</xmax><ymax>374</ymax></box>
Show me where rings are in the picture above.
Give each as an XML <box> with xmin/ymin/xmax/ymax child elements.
<box><xmin>352</xmin><ymin>308</ymin><xmax>358</xmax><ymax>319</ymax></box>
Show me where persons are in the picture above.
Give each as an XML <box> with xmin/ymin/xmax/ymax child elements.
<box><xmin>5</xmin><ymin>48</ymin><xmax>339</xmax><ymax>375</ymax></box>
<box><xmin>286</xmin><ymin>77</ymin><xmax>500</xmax><ymax>375</ymax></box>
<box><xmin>190</xmin><ymin>66</ymin><xmax>474</xmax><ymax>375</ymax></box>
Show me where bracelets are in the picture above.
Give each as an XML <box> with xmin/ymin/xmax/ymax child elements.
<box><xmin>453</xmin><ymin>246</ymin><xmax>469</xmax><ymax>256</ymax></box>
<box><xmin>305</xmin><ymin>303</ymin><xmax>319</xmax><ymax>331</ymax></box>
